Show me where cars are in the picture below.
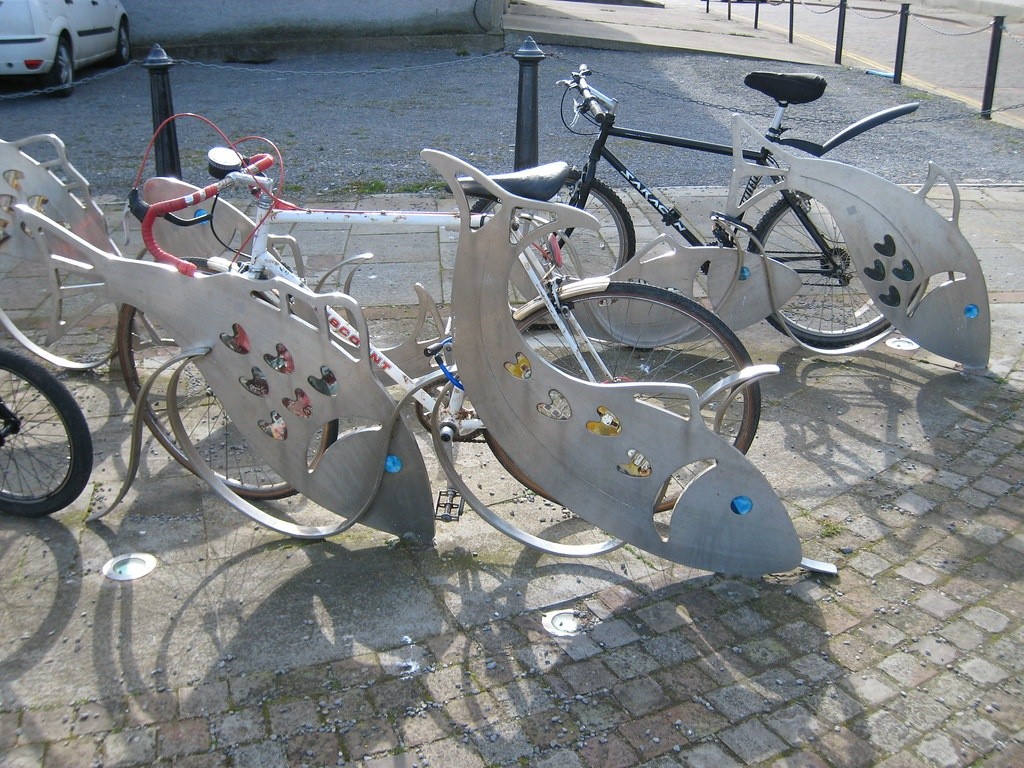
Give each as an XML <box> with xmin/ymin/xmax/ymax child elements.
<box><xmin>0</xmin><ymin>1</ymin><xmax>135</xmax><ymax>99</ymax></box>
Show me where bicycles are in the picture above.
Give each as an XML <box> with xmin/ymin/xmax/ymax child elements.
<box><xmin>117</xmin><ymin>111</ymin><xmax>764</xmax><ymax>519</ymax></box>
<box><xmin>466</xmin><ymin>63</ymin><xmax>934</xmax><ymax>351</ymax></box>
<box><xmin>0</xmin><ymin>343</ymin><xmax>96</xmax><ymax>521</ymax></box>
